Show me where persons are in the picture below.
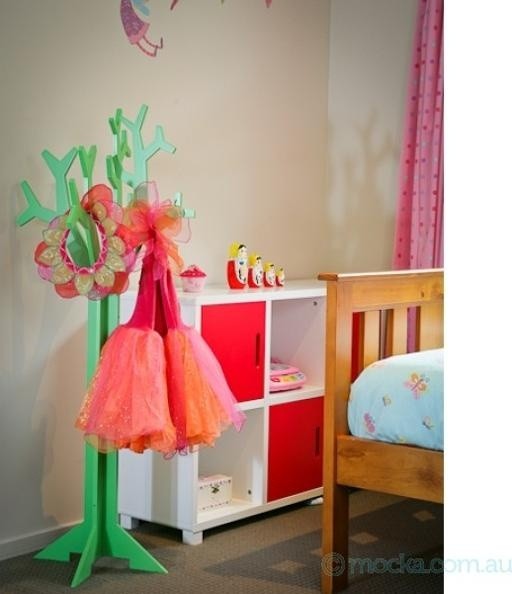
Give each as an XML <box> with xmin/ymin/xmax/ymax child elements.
<box><xmin>264</xmin><ymin>261</ymin><xmax>275</xmax><ymax>288</ymax></box>
<box><xmin>248</xmin><ymin>250</ymin><xmax>264</xmax><ymax>288</ymax></box>
<box><xmin>277</xmin><ymin>267</ymin><xmax>286</xmax><ymax>287</ymax></box>
<box><xmin>227</xmin><ymin>241</ymin><xmax>250</xmax><ymax>290</ymax></box>
<box><xmin>269</xmin><ymin>356</ymin><xmax>307</xmax><ymax>394</ymax></box>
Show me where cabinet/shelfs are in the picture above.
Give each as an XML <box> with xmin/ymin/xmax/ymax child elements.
<box><xmin>118</xmin><ymin>277</ymin><xmax>326</xmax><ymax>545</ymax></box>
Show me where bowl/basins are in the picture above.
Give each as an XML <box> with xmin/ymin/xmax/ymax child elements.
<box><xmin>180</xmin><ymin>266</ymin><xmax>208</xmax><ymax>292</ymax></box>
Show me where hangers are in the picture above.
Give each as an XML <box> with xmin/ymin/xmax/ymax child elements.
<box><xmin>15</xmin><ymin>103</ymin><xmax>169</xmax><ymax>587</ymax></box>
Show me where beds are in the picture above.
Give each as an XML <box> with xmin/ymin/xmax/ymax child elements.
<box><xmin>319</xmin><ymin>267</ymin><xmax>444</xmax><ymax>593</ymax></box>
